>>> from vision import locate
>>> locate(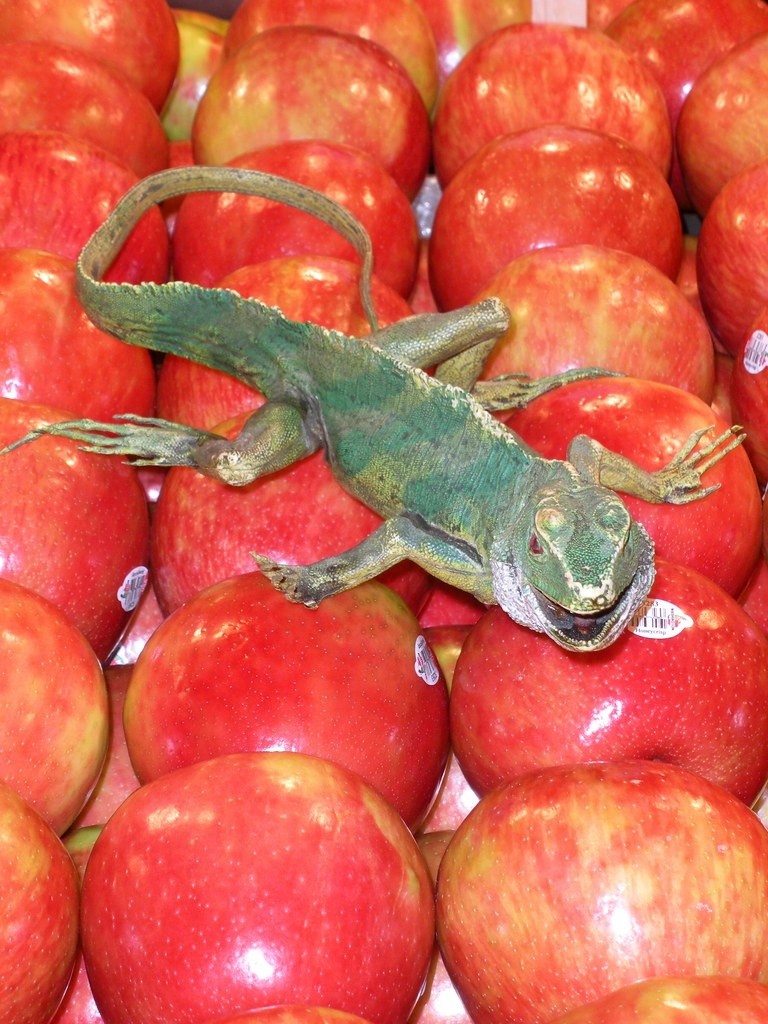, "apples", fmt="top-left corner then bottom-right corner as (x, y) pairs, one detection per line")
(0, 0), (768, 1024)
